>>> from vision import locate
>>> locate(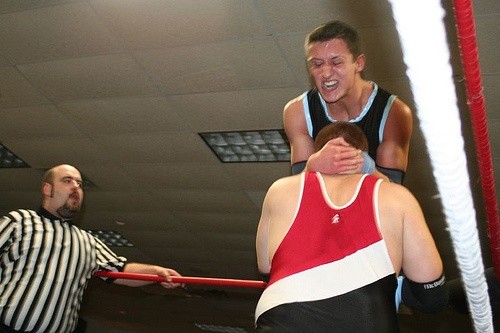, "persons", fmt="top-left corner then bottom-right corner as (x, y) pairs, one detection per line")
(255, 121), (444, 332)
(283, 20), (413, 185)
(0, 164), (185, 333)
